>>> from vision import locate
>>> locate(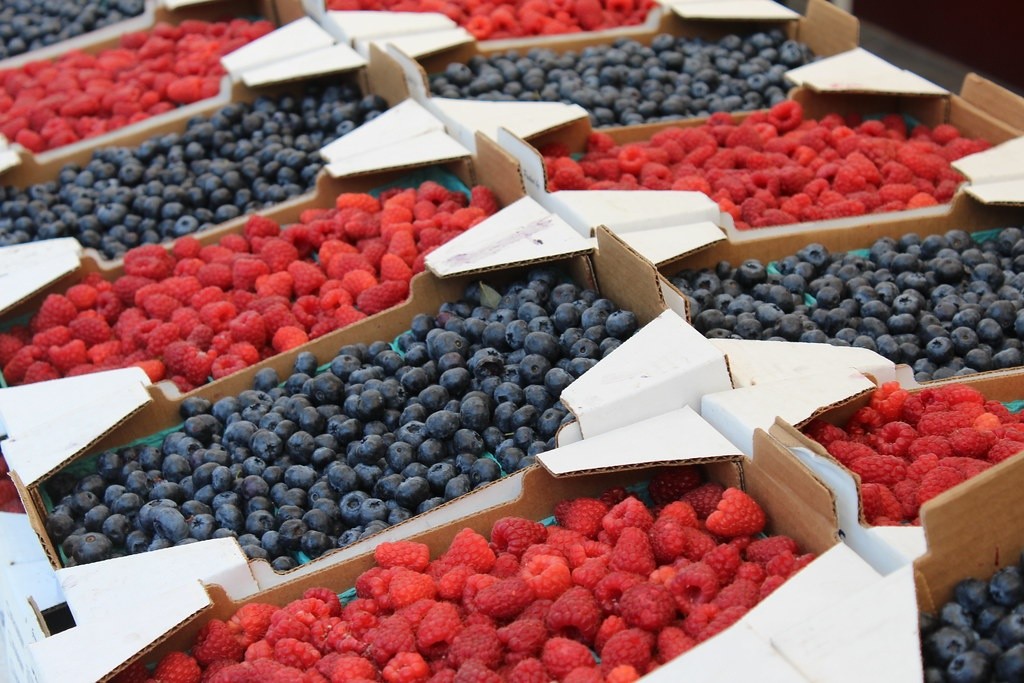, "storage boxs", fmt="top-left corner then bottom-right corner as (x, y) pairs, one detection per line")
(1, 0), (1024, 681)
